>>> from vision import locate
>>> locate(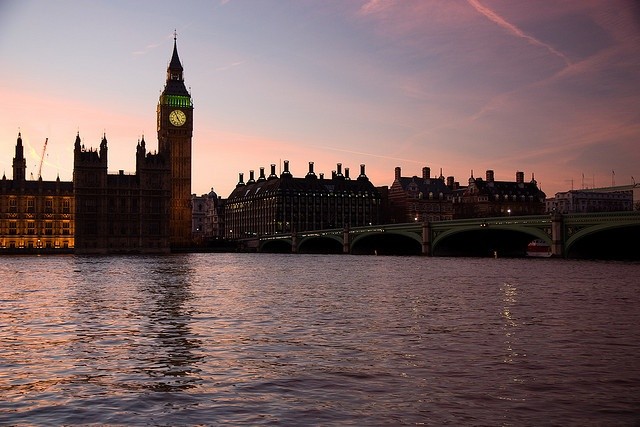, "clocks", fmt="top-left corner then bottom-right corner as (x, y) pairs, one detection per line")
(169, 109), (186, 126)
